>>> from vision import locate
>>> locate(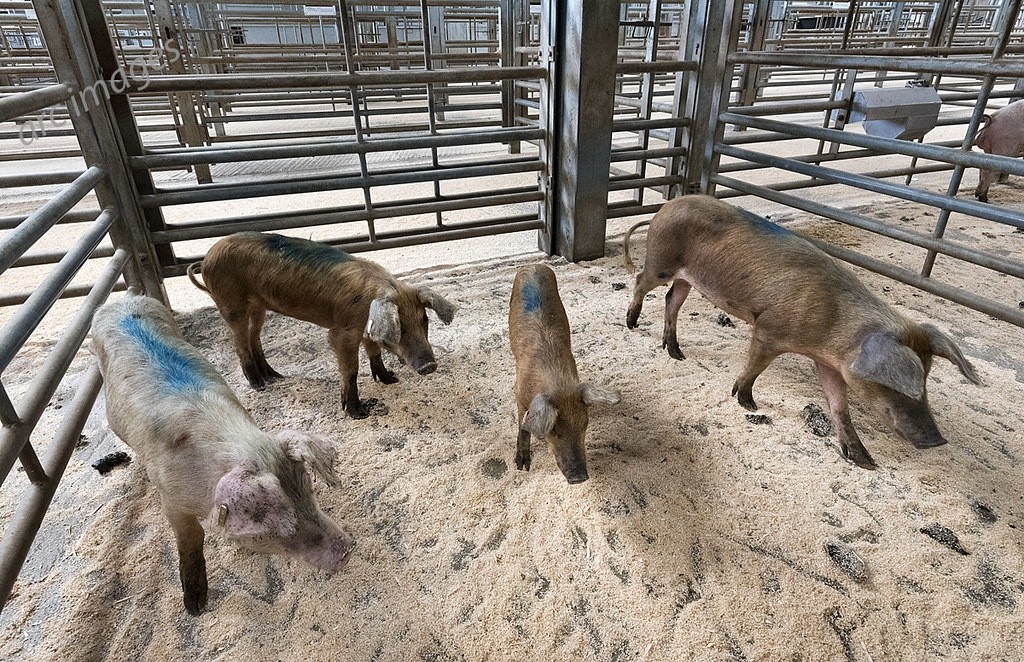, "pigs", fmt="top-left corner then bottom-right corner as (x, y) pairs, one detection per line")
(508, 265), (623, 485)
(974, 98), (1024, 203)
(623, 191), (984, 470)
(184, 231), (455, 421)
(89, 296), (356, 615)
